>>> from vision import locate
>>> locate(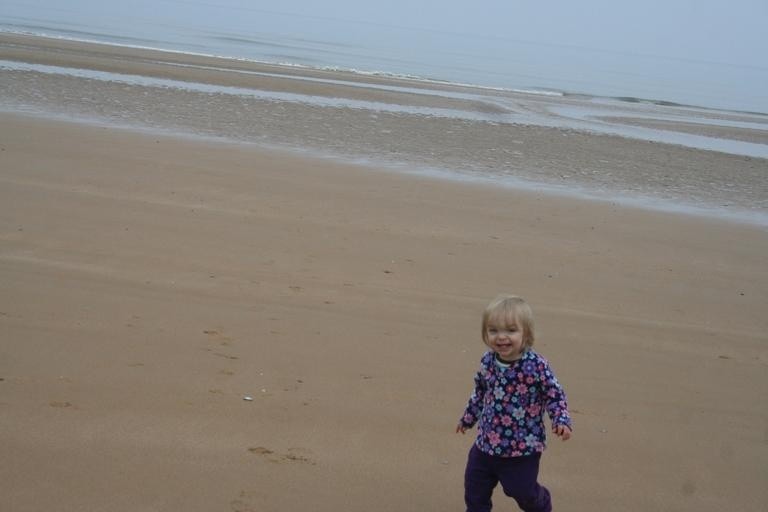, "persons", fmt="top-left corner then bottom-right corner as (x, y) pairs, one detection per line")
(456, 293), (573, 512)
